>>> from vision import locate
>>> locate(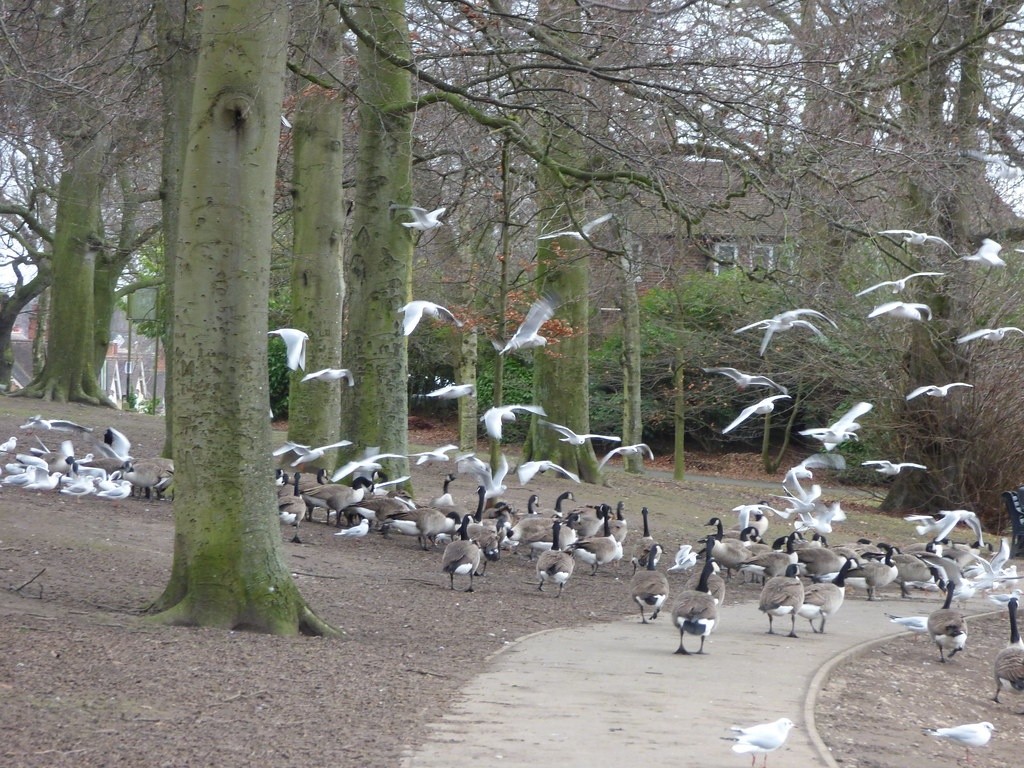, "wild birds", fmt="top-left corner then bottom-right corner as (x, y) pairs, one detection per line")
(631, 505), (660, 575)
(535, 419), (622, 446)
(989, 598), (1024, 704)
(536, 519), (575, 599)
(683, 536), (726, 606)
(630, 544), (669, 623)
(441, 515), (480, 592)
(758, 564), (802, 637)
(798, 560), (865, 636)
(598, 442), (655, 471)
(920, 721), (999, 768)
(335, 520), (370, 544)
(885, 613), (928, 644)
(40, 452), (76, 475)
(266, 202), (627, 578)
(722, 718), (798, 768)
(671, 560), (722, 652)
(0, 414), (174, 504)
(663, 226), (1023, 605)
(929, 580), (968, 663)
